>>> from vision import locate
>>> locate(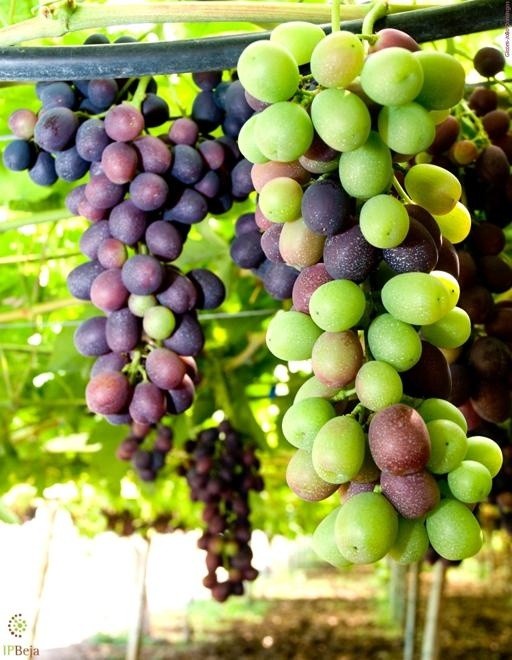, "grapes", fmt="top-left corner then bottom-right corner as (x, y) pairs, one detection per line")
(4, 21), (512, 604)
